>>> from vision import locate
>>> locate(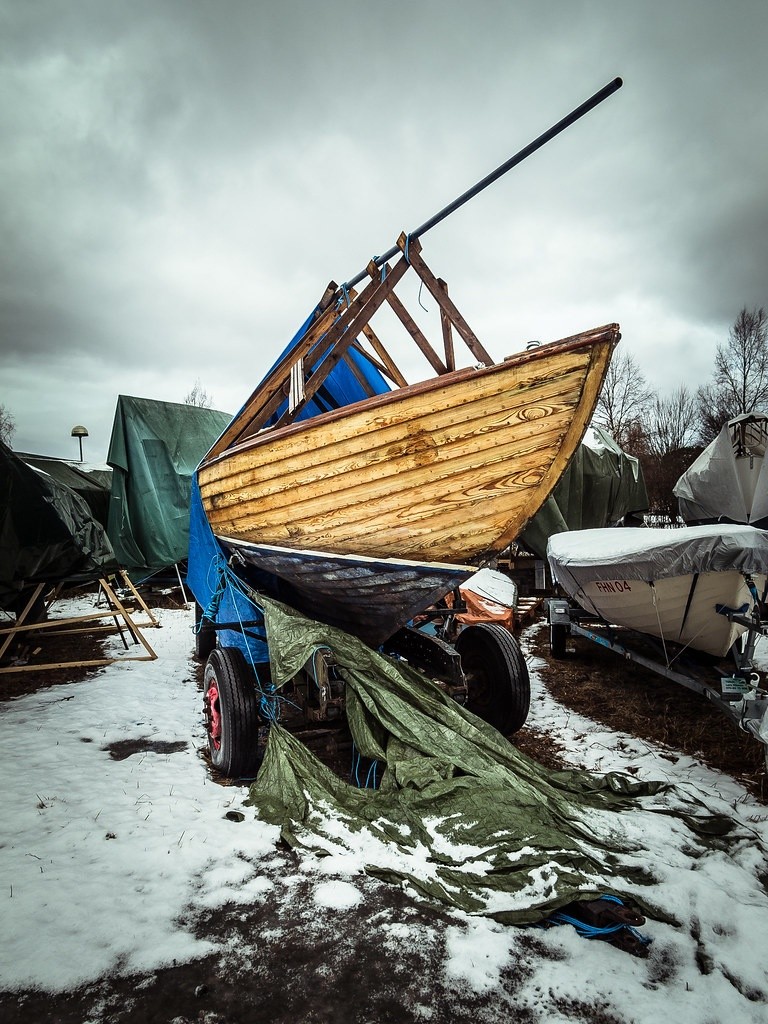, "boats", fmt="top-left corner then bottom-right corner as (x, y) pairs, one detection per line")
(193, 73), (620, 648)
(547, 526), (768, 658)
